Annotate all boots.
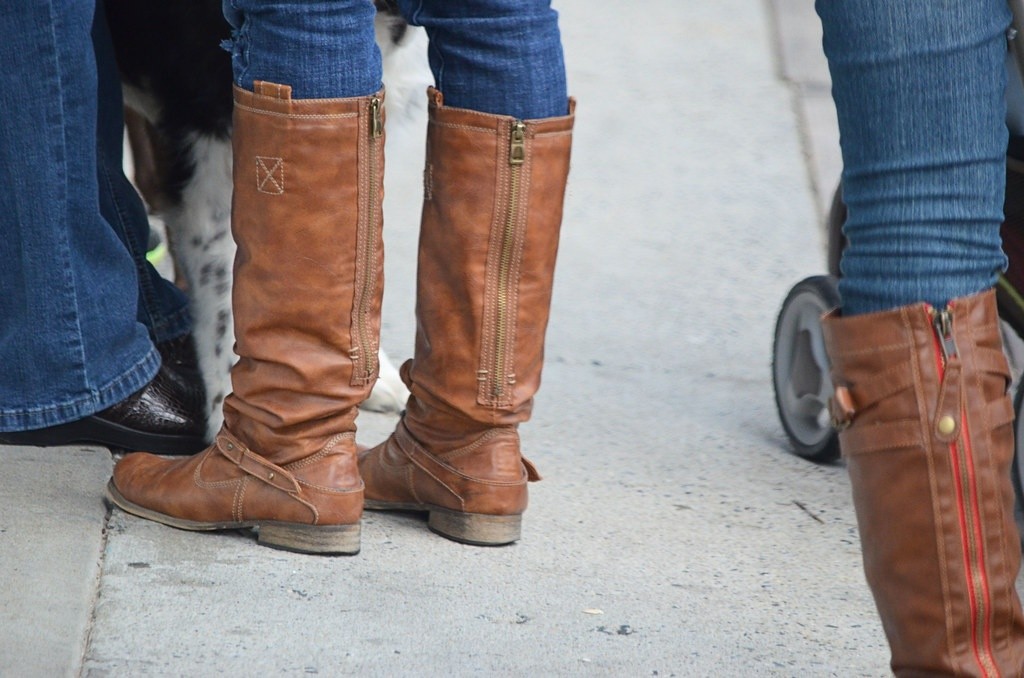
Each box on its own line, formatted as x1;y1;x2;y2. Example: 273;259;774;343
352;84;576;544
105;83;392;555
817;288;1021;673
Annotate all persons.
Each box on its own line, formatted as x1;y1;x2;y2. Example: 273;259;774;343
105;0;578;558
807;0;1024;678
0;0;209;457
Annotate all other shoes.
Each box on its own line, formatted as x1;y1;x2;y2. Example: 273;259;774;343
154;335;204;370
0;365;209;456
